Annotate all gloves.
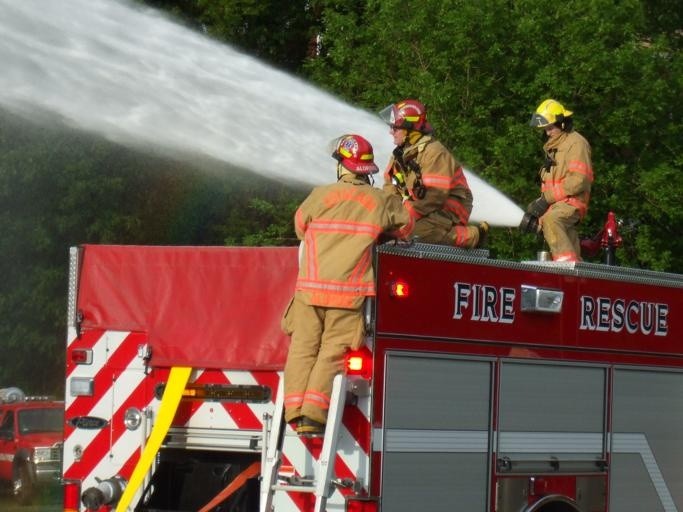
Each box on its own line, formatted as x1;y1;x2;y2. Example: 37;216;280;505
526;192;549;219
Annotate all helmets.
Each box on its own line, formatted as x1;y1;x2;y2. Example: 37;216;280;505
387;99;433;134
534;98;575;129
335;133;380;176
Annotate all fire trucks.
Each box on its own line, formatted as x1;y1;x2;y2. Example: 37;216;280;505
0;387;64;512
63;211;683;511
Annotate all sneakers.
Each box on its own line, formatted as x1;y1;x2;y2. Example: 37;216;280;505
474;221;489;250
294;415;325;433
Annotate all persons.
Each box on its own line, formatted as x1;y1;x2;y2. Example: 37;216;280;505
377;98;489;253
279;133;415;431
525;98;593;265
